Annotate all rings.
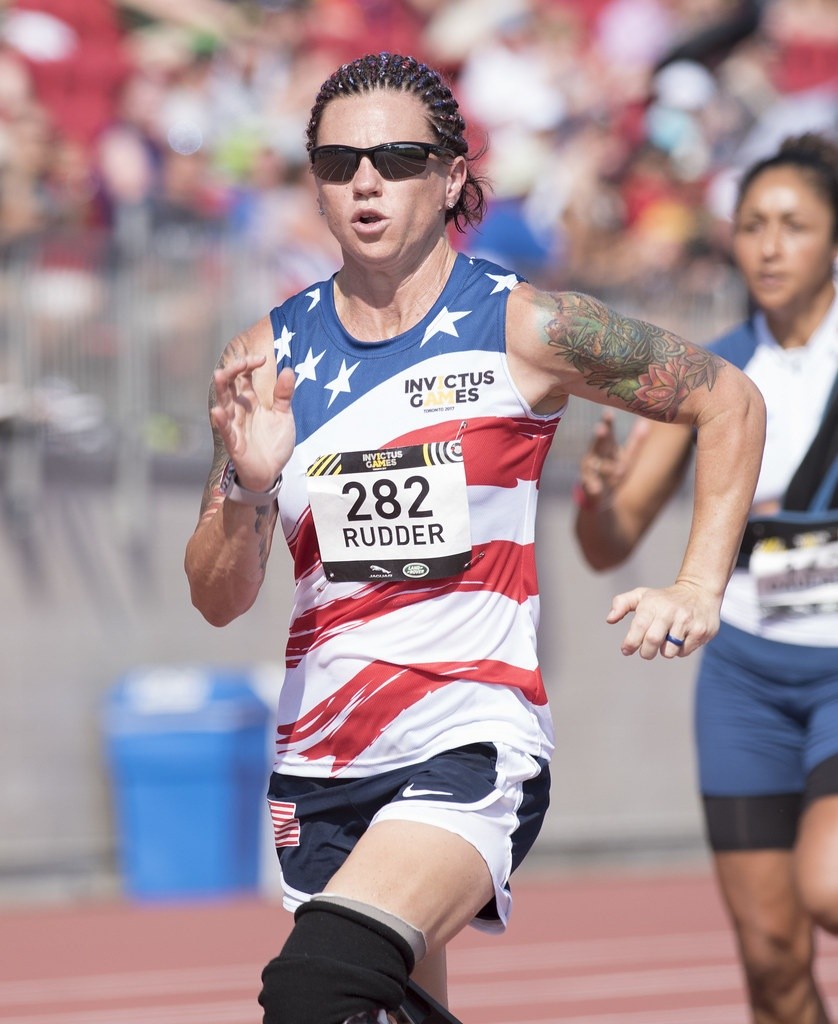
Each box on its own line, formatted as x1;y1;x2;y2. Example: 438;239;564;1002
666;634;683;646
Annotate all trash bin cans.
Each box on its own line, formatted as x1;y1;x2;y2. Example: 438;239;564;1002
103;664;273;903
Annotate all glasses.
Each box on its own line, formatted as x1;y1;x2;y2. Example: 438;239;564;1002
309;140;459;181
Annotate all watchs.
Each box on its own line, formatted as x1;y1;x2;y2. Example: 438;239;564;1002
218;457;283;505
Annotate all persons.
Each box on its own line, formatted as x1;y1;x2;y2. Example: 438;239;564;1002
185;51;766;1024
576;133;837;1024
